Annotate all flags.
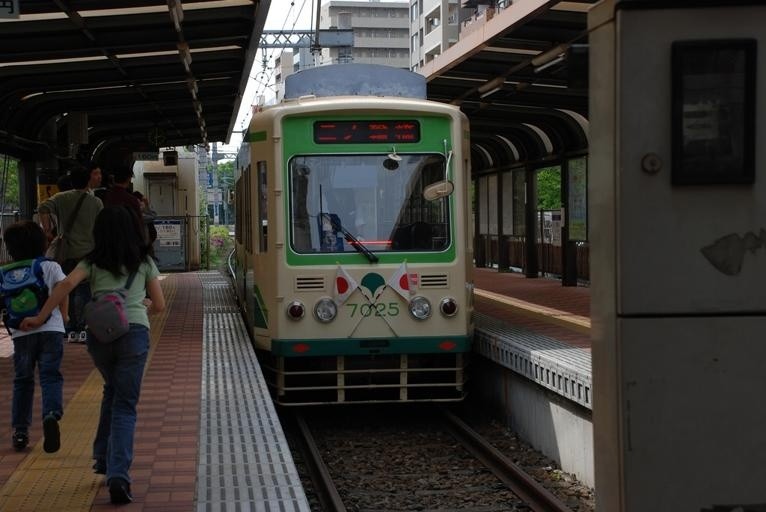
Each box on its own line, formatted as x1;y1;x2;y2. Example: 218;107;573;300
387;261;417;304
333;265;358;306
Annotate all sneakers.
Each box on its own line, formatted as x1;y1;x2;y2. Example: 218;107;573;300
67;329;88;344
12;431;29;452
43;414;60;453
93;458;132;503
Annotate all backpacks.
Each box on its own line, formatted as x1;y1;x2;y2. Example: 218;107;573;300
0;259;51;328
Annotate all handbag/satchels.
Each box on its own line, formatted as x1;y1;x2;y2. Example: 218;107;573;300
45;234;65;265
83;289;131;345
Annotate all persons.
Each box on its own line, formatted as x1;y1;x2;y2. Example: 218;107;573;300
0;220;72;453
89;167;161;264
37;167;104;344
18;205;167;502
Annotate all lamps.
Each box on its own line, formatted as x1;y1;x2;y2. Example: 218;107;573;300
449;27;569;106
166;0;212;153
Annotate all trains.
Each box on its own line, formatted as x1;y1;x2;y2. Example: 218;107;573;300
235;61;480;410
